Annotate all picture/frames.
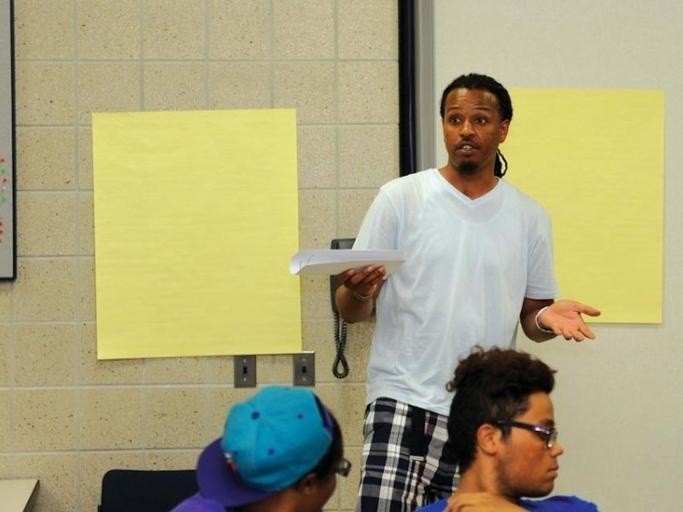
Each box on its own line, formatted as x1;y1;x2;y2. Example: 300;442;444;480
0;0;16;282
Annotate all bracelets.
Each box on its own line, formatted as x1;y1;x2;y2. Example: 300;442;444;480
534;305;562;334
352;292;372;303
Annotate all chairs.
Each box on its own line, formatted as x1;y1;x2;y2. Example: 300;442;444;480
96;469;201;512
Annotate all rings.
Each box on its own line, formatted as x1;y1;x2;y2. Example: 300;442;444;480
343;279;357;289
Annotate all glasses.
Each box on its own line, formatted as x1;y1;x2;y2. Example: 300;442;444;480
487;418;559;449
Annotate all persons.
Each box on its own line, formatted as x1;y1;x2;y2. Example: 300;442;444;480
169;385;351;512
413;343;599;512
335;74;603;510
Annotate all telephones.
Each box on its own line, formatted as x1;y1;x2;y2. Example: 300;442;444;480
330;239;376;378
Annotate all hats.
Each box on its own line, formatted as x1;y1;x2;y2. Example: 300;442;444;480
197;383;337;506
